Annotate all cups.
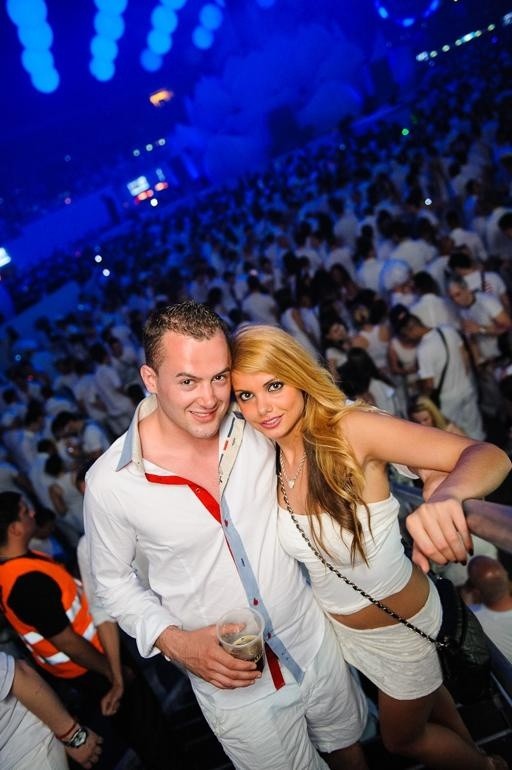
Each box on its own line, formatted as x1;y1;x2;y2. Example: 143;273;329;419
216;607;266;672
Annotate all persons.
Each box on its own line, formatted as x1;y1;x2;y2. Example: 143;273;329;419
0;36;512;558
0;490;182;770
464;553;512;662
76;300;370;770
227;324;512;770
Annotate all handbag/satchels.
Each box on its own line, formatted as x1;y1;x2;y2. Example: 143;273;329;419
412;392;440;409
433;578;490;692
